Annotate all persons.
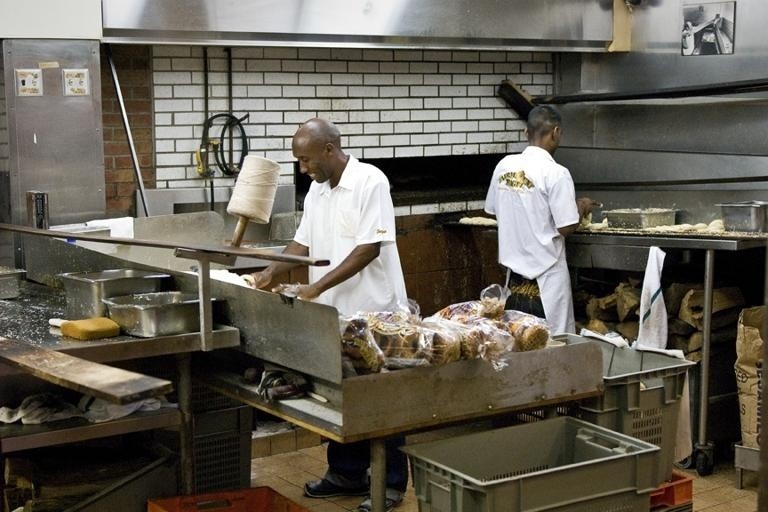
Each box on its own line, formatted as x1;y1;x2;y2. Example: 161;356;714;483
248;118;408;512
683;15;721;55
484;106;600;339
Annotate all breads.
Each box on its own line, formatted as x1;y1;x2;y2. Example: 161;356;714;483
343;319;548;374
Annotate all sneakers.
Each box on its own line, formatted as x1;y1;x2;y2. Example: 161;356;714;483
303;478;370;499
358;497;398;511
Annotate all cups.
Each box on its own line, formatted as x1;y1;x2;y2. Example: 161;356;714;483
26;190;48;229
